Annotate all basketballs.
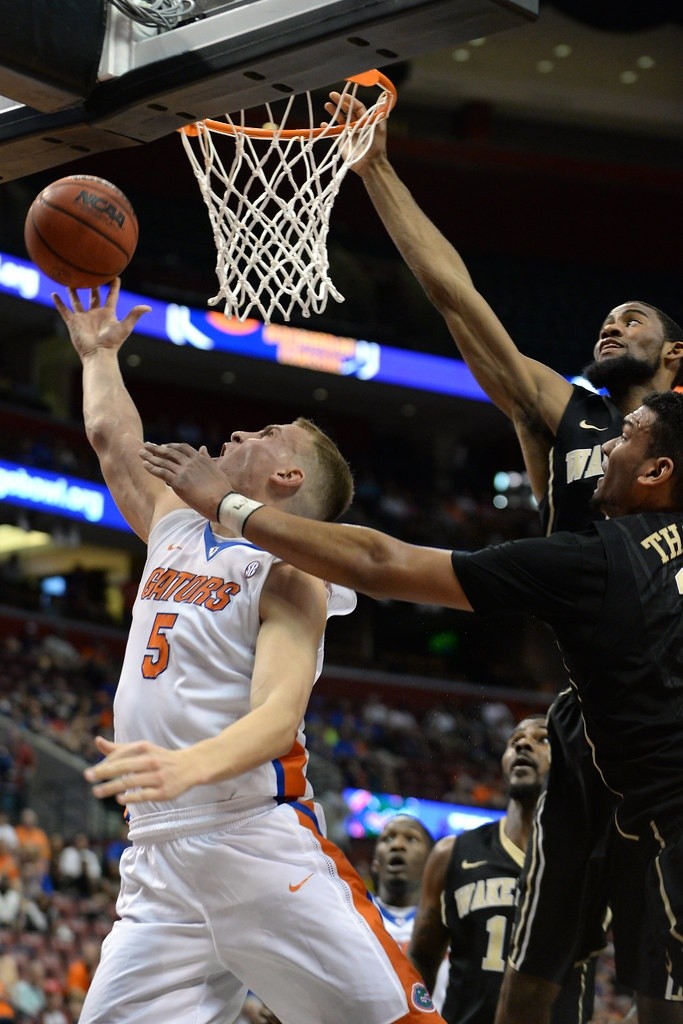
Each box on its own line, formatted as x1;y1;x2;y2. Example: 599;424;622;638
23;173;140;290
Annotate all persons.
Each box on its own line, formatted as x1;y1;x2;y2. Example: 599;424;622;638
137;392;683;1024
318;93;683;539
51;279;446;1024
367;813;451;1016
0;605;683;1024
408;715;597;1024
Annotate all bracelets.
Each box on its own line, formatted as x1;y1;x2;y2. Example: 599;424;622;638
217;491;265;539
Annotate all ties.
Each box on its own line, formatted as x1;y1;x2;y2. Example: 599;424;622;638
77;847;87;876
